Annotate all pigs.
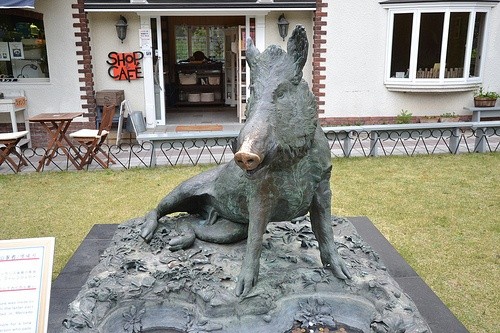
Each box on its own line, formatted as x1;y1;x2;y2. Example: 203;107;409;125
138;22;352;298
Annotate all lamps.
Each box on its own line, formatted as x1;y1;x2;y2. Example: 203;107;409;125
114;13;128;43
277;12;290;40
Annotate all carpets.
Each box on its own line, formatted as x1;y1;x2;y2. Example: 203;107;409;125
176;125;222;131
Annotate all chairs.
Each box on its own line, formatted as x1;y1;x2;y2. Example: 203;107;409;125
0;130;29;173
68;103;117;169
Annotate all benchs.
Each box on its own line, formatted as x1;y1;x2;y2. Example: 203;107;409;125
463;106;500;121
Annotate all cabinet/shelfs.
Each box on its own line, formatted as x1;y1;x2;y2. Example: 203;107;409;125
175;61;225;106
224;24;256;123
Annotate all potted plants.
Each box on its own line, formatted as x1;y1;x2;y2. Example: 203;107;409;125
474;86;500;107
439;111;459;121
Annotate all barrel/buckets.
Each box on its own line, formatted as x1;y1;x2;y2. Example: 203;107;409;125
126;112;147;132
201;93;214;102
189;94;200;102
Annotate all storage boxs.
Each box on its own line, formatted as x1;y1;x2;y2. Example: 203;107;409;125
179;73;197;84
96;89;124;106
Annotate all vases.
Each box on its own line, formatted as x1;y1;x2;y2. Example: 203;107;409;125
419;117;439;122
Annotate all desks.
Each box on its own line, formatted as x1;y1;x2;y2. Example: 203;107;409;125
25;112;86;171
0;95;31;154
